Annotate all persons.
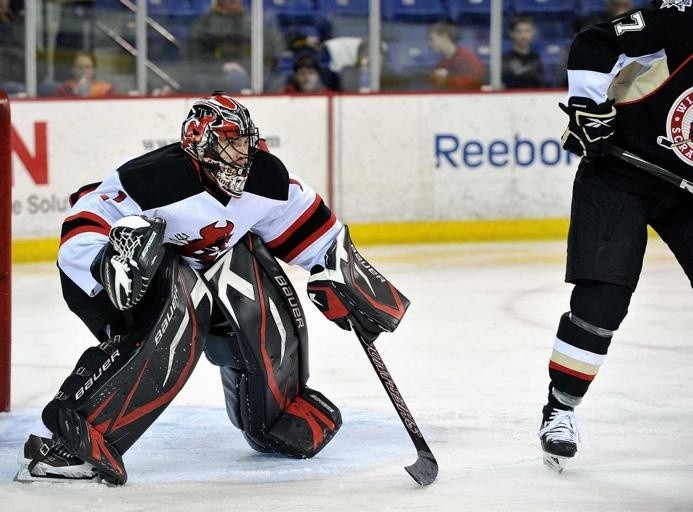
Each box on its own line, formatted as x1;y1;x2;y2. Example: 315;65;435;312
1;1;24;28
536;0;693;460
191;0;341;94
57;51;116;97
15;89;411;474
428;18;544;90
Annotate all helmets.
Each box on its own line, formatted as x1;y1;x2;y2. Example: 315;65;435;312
180;93;260;199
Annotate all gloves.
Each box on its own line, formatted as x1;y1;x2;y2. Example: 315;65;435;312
558;97;626;166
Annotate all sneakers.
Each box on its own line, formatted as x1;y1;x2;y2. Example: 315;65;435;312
17;435;101;478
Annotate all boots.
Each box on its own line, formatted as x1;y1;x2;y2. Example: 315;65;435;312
540;381;586;458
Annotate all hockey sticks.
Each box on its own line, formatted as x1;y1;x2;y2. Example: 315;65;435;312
354;327;439;486
